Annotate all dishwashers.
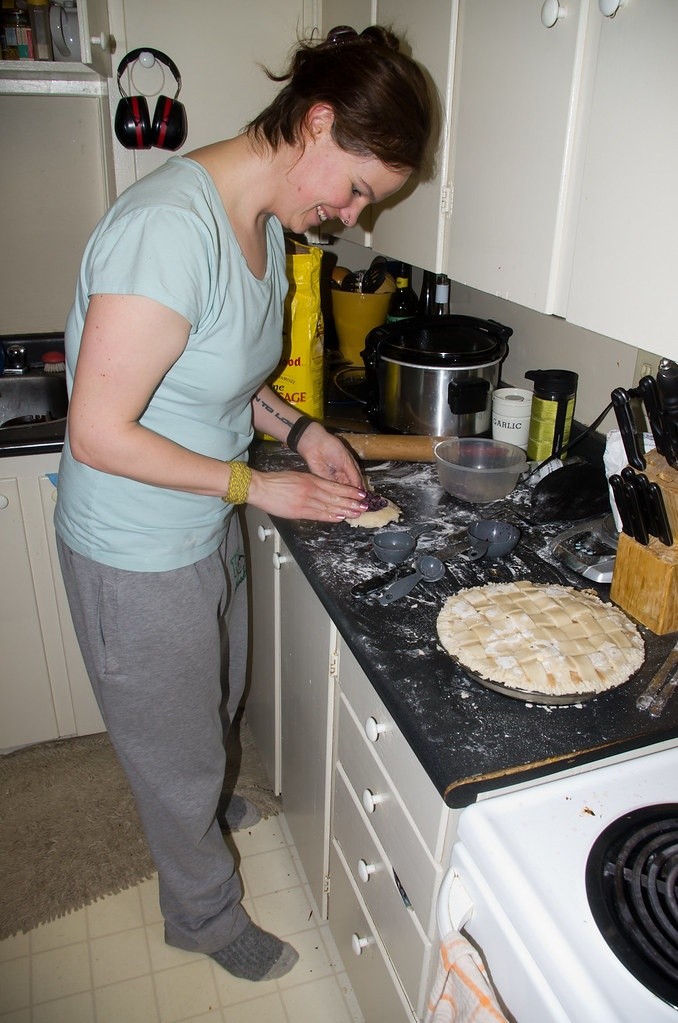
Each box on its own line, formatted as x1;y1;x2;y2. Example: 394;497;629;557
422;841;571;1023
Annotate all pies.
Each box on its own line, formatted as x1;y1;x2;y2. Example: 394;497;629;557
436;579;646;696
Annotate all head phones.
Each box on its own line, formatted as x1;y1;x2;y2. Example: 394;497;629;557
113;47;188;151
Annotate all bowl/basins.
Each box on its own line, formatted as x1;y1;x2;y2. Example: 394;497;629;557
433;438;530;503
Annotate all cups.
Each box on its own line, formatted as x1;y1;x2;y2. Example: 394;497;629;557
492;386;532;453
50;1;81;62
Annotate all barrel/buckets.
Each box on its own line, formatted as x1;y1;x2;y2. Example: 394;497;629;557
330;287;392;368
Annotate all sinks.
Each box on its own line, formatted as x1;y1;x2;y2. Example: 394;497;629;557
0;375;71;438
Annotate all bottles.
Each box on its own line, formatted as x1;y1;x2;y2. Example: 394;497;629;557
526;369;577;460
387;262;449;321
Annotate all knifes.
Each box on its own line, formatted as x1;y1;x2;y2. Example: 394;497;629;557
350;540;472;598
610;357;678;546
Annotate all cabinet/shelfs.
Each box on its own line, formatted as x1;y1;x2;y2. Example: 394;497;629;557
0;474;60;752
442;1;677;364
38;477;107;737
239;503;337;920
124;1;320;244
320;1;458;274
327;629;449;1022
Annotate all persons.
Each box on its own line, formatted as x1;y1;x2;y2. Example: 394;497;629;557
54;26;443;983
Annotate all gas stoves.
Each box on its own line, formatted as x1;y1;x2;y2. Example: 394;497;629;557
456;734;678;1023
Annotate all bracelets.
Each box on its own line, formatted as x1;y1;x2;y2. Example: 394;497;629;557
287;415;317;453
221;460;251;505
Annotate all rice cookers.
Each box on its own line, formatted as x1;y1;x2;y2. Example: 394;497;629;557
360;315;513;436
